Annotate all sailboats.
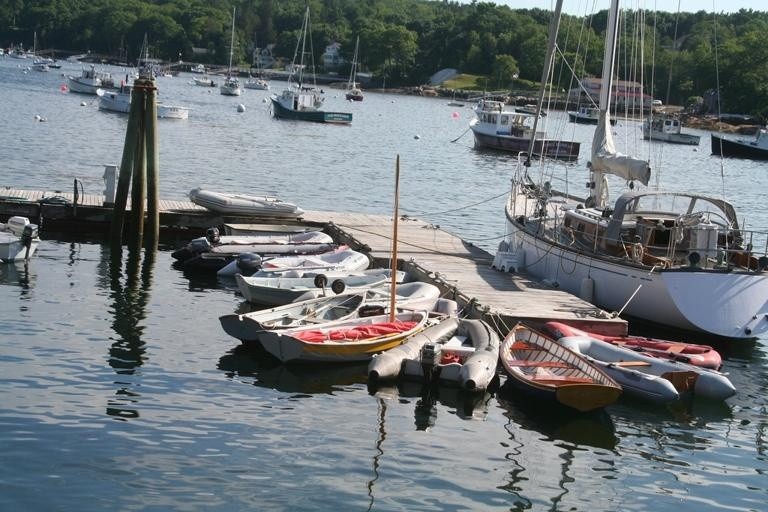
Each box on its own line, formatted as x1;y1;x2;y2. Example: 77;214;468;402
505;0;766;341
346;35;364;101
283;6;325;108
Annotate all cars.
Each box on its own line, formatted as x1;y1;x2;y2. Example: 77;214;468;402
653;100;662;105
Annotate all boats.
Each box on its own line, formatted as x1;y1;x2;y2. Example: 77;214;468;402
639;108;700;145
514;104;547;119
367;315;501;393
0;216;40;262
182;236;341;264
545;320;723;372
270;92;353;123
192;76;219;87
31;62;50;71
468;99;581;164
189;187;305;218
216;248;369;279
500;321;624;412
218;282;440;343
26;50;35;58
170;231;339;260
251;250;368;278
10;46;27;58
68;62;122;95
710;126;768;161
223;223;326;237
32;55;54;64
232;273;394;307
556;336;738;404
568;104;618;126
221;72;241;95
256;309;429;364
244;77;270;90
45;61;61;69
96;30;189;119
